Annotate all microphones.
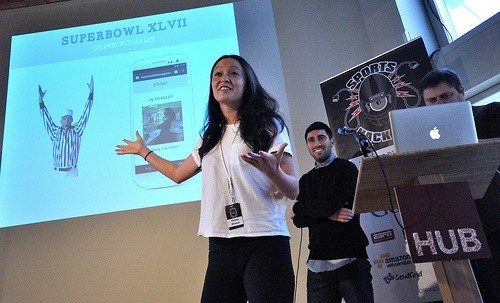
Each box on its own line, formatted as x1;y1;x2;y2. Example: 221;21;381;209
341;124;348;136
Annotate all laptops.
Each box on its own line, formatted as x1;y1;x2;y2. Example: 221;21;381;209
389;100;479;153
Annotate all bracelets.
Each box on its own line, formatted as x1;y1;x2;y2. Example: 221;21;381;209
145;151;154;161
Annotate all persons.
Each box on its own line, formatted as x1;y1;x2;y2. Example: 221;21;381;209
38;75;94;178
116;55;300;303
418;69;500;303
290;122;374;303
